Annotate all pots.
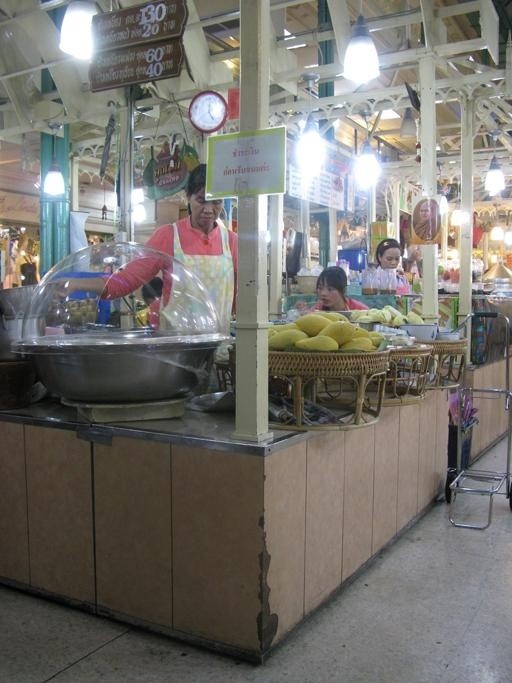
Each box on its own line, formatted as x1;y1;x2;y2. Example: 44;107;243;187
0;313;42;361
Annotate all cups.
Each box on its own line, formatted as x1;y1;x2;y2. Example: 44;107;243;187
380;270;397;294
361;271;378;295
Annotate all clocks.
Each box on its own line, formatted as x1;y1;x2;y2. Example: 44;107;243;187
189;90;228;133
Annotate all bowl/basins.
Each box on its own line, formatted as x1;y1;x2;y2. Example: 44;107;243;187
0;282;39;315
297;274;319;294
399;322;439;344
437;329;462;344
10;325;229;404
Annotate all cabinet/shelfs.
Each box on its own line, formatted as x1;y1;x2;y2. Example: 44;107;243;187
1;351;451;655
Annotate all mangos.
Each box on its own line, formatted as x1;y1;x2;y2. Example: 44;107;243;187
268;310;386;353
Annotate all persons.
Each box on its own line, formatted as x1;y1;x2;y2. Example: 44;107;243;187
309;267;368;312
15;250;42;288
362;238;411;295
134;278;166;325
413;201;439;239
47;164;239;335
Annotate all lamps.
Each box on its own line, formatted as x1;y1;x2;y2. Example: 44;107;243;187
484;139;504;196
399;110;418;141
451;176;467;226
437;166;449;216
504;230;512;247
342;1;380;84
59;2;101;62
353;118;382;190
43;135;65;197
295;88;325;169
133;190;147;223
490;204;504;241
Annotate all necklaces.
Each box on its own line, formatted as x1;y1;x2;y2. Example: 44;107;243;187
23;255;33;265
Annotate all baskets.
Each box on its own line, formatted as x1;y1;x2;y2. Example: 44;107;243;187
367;347;434;409
269;349;391;431
418;339;469;392
